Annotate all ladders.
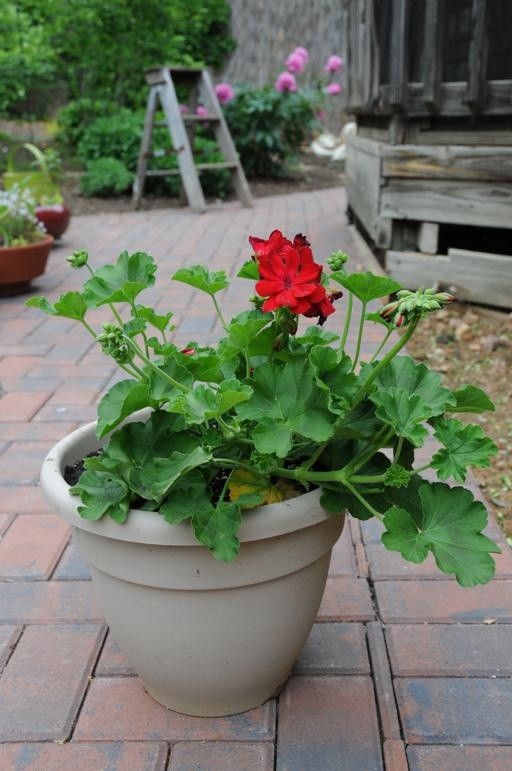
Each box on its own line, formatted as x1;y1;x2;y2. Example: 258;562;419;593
130;64;255;215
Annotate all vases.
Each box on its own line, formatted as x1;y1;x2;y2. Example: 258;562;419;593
39;405;346;719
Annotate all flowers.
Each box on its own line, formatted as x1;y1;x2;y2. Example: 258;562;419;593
166;45;344;199
20;225;503;589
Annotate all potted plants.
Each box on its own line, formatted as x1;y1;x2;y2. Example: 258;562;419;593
0;143;71;297
